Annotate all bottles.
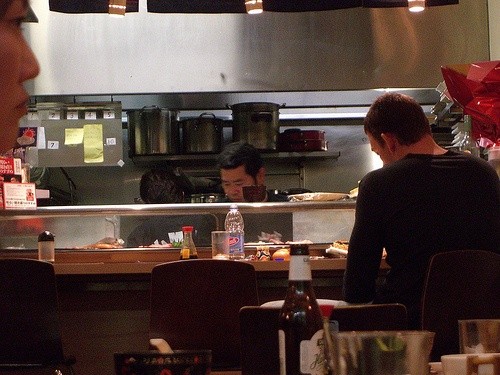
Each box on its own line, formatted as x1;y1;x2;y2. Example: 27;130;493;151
279;244;330;374
458;114;500;182
179;226;198;259
38;231;55;264
225;204;245;259
251;246;290;260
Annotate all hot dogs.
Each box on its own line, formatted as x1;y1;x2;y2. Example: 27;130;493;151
332;240;349;250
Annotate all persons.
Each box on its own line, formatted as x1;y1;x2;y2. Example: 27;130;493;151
338;90;500;358
130;141;296;246
0;174;20;190
0;0;42;152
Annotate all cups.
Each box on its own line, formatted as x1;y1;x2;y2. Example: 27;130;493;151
332;330;434;375
211;231;230;260
430;319;500;375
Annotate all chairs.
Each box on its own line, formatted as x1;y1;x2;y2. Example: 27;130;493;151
236;304;411;375
150;259;260;352
421;251;500;349
0;258;63;367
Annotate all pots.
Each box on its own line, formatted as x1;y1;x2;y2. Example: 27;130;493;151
126;104;178;156
226;101;286;151
179;111;234;153
280;128;328;152
189;192;228;202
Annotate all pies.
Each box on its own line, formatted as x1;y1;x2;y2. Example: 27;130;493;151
94;243;122;249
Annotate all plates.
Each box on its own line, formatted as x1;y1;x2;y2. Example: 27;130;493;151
288;190;347;201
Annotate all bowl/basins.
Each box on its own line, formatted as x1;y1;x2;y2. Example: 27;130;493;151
242;184;267;202
268;189;288;202
112;350;213;375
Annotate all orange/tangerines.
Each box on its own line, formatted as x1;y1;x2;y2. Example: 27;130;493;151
272;249;290;260
